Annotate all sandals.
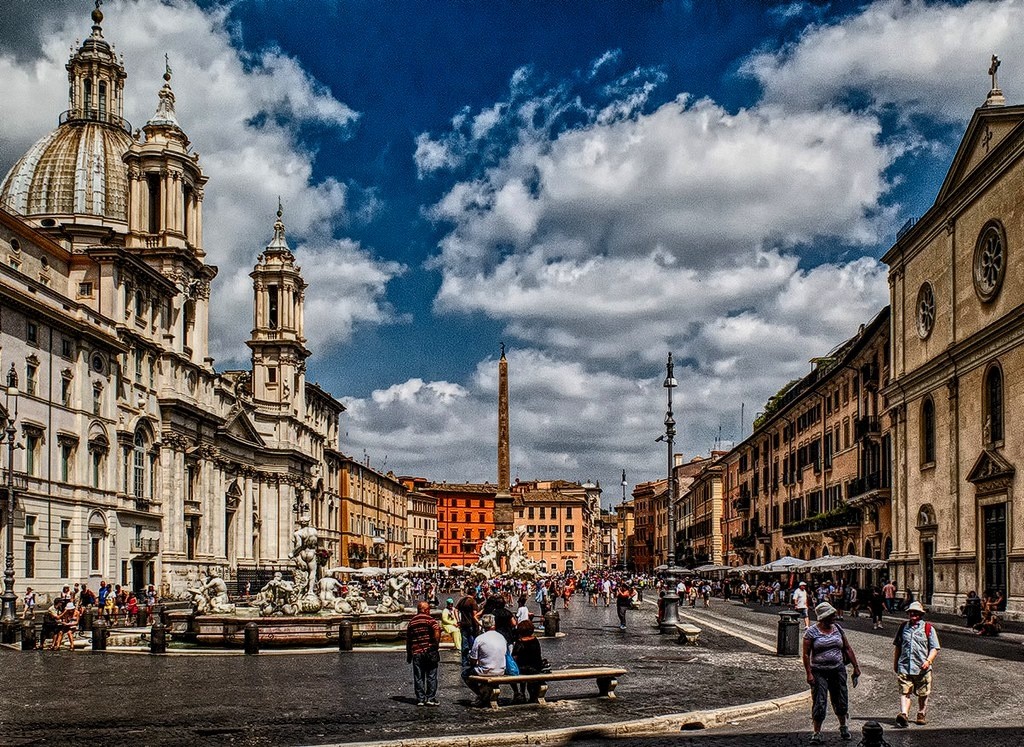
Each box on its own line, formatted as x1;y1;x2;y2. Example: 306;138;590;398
11;615;159;651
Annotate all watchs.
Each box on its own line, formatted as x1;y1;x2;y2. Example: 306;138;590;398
852;664;860;668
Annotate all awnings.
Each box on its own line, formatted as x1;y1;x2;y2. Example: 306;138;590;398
653;549;888;586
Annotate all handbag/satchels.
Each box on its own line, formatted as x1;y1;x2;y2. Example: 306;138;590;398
408;622;440;662
505;650;519;676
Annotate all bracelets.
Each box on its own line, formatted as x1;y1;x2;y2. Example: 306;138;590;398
925;658;932;666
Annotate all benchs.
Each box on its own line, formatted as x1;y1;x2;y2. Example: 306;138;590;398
673;623;702;646
510;609;560;637
467;666;628;712
3;612;447;655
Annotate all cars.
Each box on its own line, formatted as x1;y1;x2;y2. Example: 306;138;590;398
347;581;362;589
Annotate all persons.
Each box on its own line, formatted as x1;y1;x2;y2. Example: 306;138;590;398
474;523;540;581
404;601;444;706
254;512;412;613
980;592;993;612
961;589;981;627
987;590;1006;611
891;599;941;726
458;611;511;710
324;565;915;664
244;579;251;600
200;568;240;615
507;616;546;708
972;609;1002;637
801;601;861;746
20;577;161;653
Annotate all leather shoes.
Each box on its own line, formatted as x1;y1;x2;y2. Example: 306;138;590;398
896;712;908;726
916;710;926;725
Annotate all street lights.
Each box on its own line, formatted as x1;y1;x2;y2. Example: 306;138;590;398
386;505;391;581
0;361;27;630
540;541;544;572
653;351;692;635
621;468;628;581
461;532;466;571
292;500;310;517
436;525;440;574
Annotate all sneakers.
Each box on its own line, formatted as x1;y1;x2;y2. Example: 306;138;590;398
511;692;522;703
418;697;425;705
810;731;824;745
838;725;851;740
470;695;484;706
518;694;527;703
426;696;441;705
619;623;627;629
538;625;546;629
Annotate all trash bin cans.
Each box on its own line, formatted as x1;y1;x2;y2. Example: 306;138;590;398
777;610;801;657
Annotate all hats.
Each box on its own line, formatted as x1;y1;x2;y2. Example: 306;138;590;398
815;602;837;621
905;601;926;615
799;582;807;586
